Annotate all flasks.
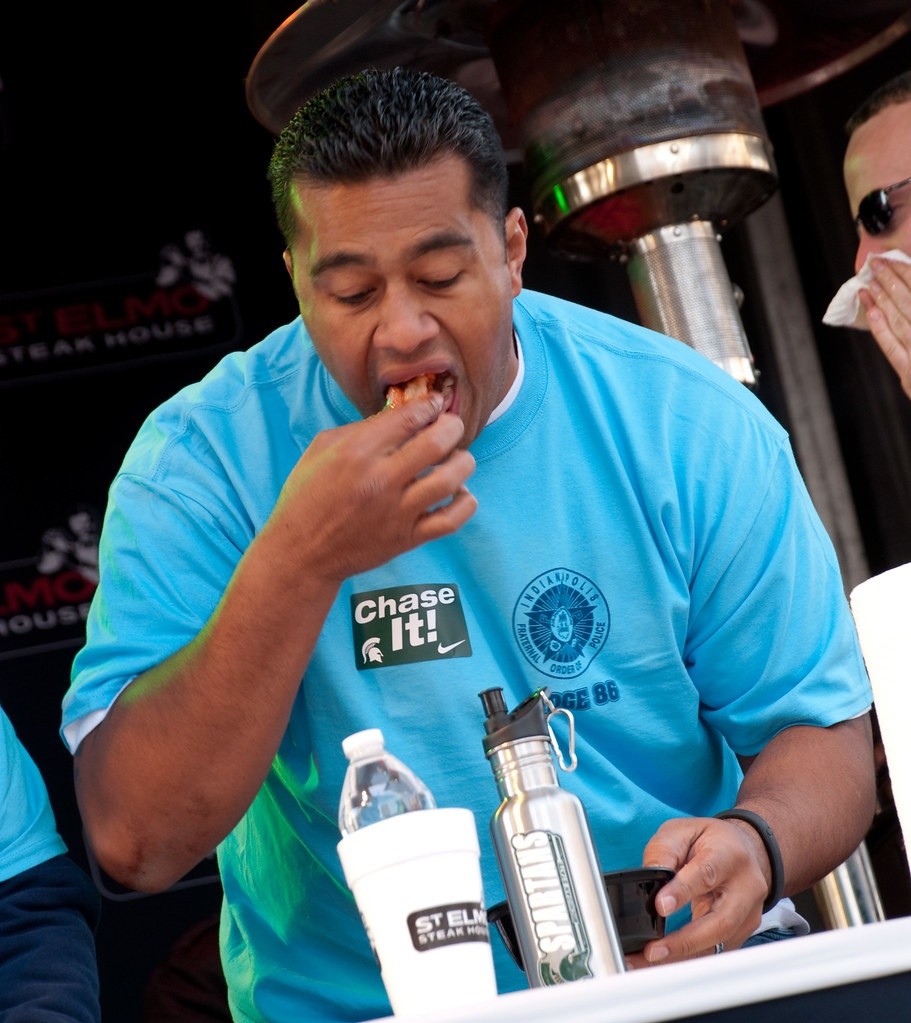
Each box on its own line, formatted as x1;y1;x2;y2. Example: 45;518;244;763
477;681;626;988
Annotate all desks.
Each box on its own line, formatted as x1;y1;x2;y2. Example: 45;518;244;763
360;912;911;1023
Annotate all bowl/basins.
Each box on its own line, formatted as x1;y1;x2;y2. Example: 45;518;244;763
486;868;673;972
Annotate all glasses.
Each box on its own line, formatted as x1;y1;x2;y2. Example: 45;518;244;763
853;176;911;236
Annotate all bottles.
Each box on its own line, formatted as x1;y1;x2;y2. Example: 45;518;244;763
336;730;435;839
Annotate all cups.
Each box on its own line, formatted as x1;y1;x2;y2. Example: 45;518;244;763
335;806;496;1023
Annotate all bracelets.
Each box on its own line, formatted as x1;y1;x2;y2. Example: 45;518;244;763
713;808;785;914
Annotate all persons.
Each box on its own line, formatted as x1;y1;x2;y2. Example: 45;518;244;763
0;707;100;1023
841;68;911;403
54;60;877;1023
